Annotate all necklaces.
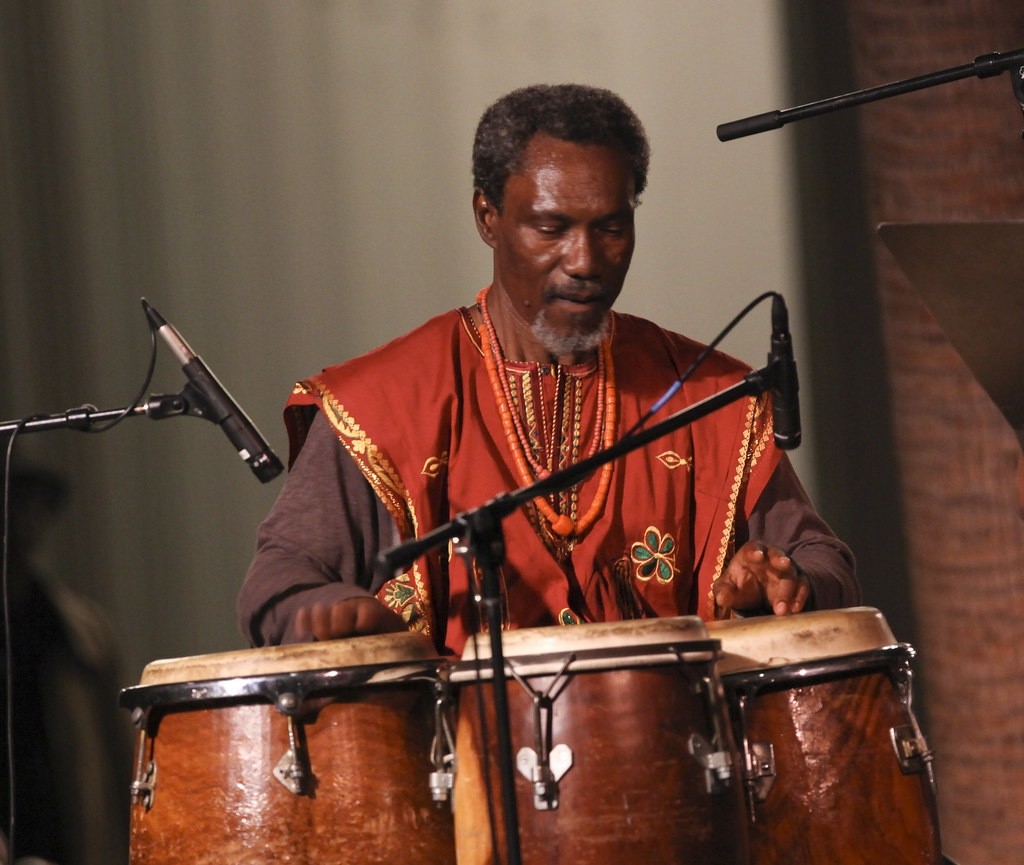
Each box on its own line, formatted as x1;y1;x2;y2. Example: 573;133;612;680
477;285;618;563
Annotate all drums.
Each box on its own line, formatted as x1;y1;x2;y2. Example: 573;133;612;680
127;631;454;865
450;615;755;865
704;605;945;865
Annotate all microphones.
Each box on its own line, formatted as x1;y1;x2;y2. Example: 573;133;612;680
145;304;284;484
769;294;801;451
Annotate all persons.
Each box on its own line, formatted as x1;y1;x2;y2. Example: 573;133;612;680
235;85;861;648
